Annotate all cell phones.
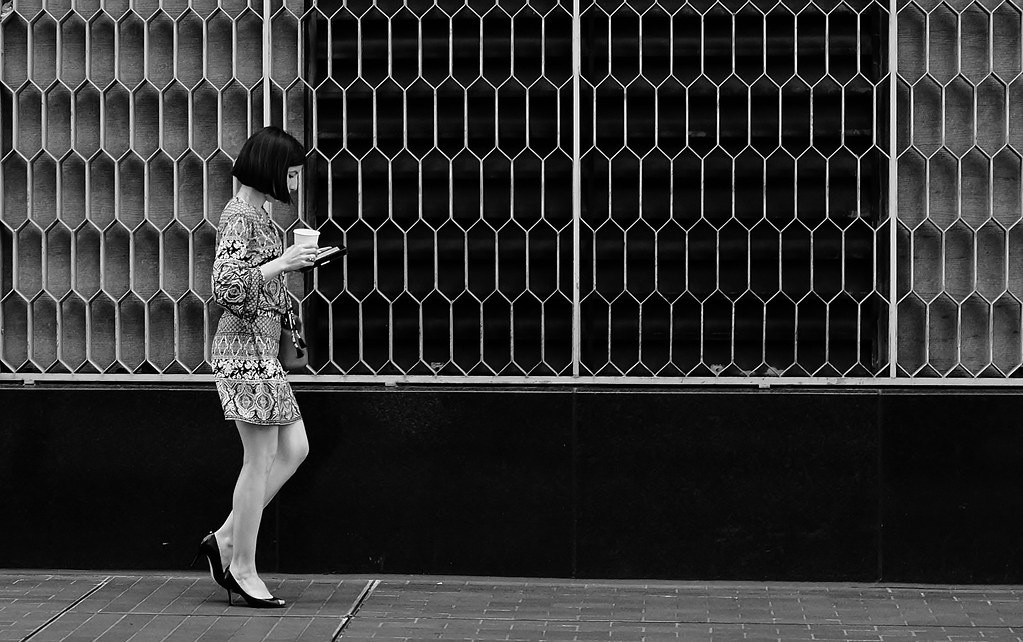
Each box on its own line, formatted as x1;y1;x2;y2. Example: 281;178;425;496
300;244;348;273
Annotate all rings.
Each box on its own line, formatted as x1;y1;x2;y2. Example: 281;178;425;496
308;254;313;260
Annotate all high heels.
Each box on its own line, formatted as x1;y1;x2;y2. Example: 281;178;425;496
190;531;226;589
223;569;286;608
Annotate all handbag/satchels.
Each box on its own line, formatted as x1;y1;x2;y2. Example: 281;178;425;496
278;325;308;370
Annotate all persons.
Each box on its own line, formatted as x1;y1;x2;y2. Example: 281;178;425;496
193;124;321;607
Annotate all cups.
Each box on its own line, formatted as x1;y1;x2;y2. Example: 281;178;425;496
293;228;320;250
